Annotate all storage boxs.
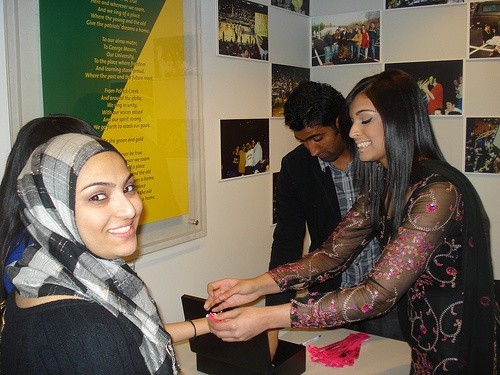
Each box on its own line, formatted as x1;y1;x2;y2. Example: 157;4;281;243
180;295;306;375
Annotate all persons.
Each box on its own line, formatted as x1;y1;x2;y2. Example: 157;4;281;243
1;132;179;375
0;115;227;344
204;67;500;375
265;79;407;360
219;0;500;177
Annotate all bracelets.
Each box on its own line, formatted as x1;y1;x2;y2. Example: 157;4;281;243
190;320;196;339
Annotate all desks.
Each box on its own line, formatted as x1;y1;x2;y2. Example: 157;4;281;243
172;326;413;375
